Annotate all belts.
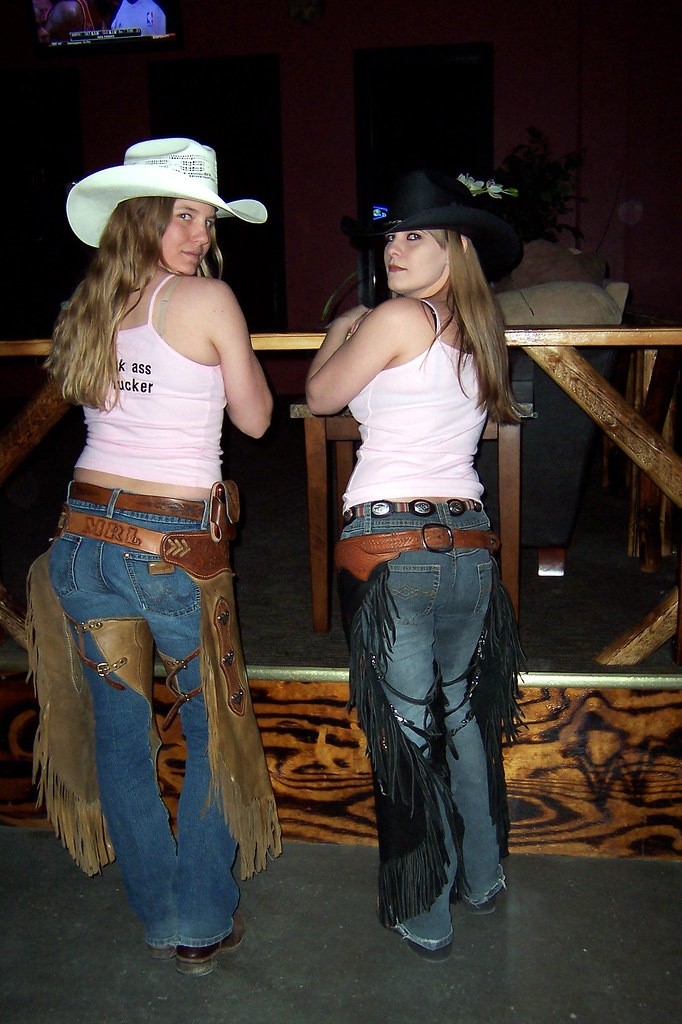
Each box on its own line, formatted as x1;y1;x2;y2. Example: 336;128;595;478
326;526;498;581
68;480;206;521
54;504;231;578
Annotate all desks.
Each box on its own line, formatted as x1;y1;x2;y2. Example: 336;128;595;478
287;379;537;638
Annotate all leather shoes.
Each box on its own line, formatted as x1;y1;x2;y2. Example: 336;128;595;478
176;910;248;975
375;899;453;963
464;896;497;914
147;941;177;959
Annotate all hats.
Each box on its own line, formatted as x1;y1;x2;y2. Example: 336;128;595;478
66;138;269;248
338;171;525;284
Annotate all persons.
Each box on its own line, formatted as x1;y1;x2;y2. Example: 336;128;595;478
46;130;278;974
305;160;531;956
